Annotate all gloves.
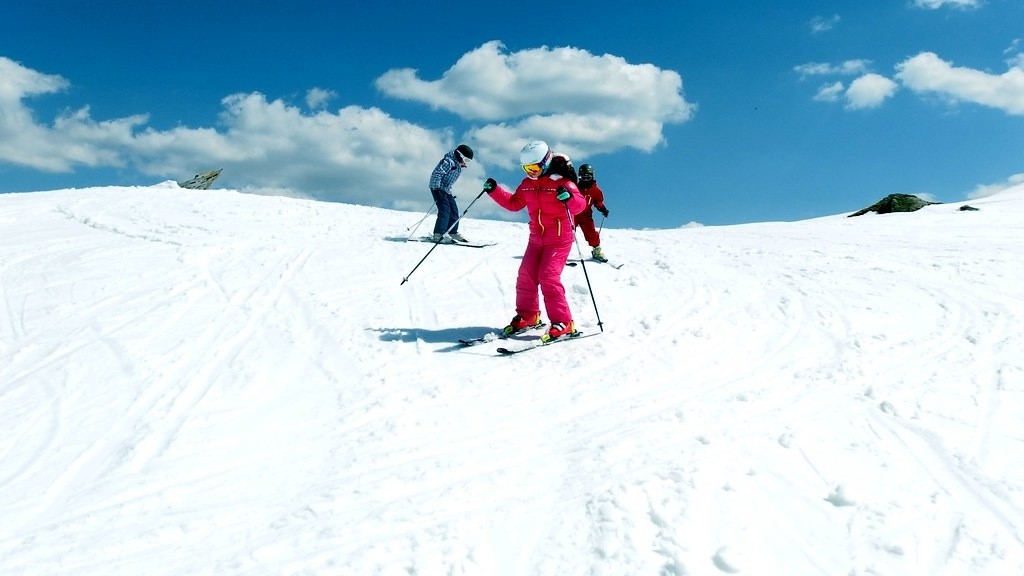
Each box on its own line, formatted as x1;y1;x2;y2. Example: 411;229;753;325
556;186;573;202
433;190;442;200
600;206;609;217
484;178;497;194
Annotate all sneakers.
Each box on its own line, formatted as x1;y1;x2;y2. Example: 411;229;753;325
502;314;542;335
434;232;457;244
593;247;608;262
448;234;468;243
542;320;577;342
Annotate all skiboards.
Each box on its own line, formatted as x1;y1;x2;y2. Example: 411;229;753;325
568;257;623;269
457;322;581;355
386;235;498;248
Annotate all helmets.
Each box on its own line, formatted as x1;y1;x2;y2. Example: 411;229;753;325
578;164;593;177
454;145;474;163
520;140;552;166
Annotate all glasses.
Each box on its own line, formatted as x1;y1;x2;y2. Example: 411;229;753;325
457;150;472;165
580;174;592;180
523;149;551;173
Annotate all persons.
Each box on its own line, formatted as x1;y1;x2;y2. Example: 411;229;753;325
483;141;584;341
430;145;474;242
575;164;609;261
